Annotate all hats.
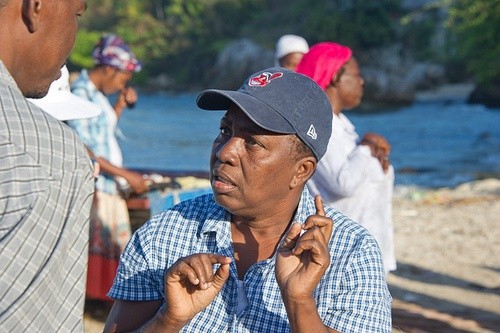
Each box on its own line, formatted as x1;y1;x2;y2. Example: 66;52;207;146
24;64;102;120
95;34;142;72
274;35;310;67
294;42;352;88
195;66;333;162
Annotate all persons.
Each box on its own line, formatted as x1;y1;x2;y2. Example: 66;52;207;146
295;42;397;272
0;0;95;333
275;35;309;71
64;32;149;265
104;66;393;333
